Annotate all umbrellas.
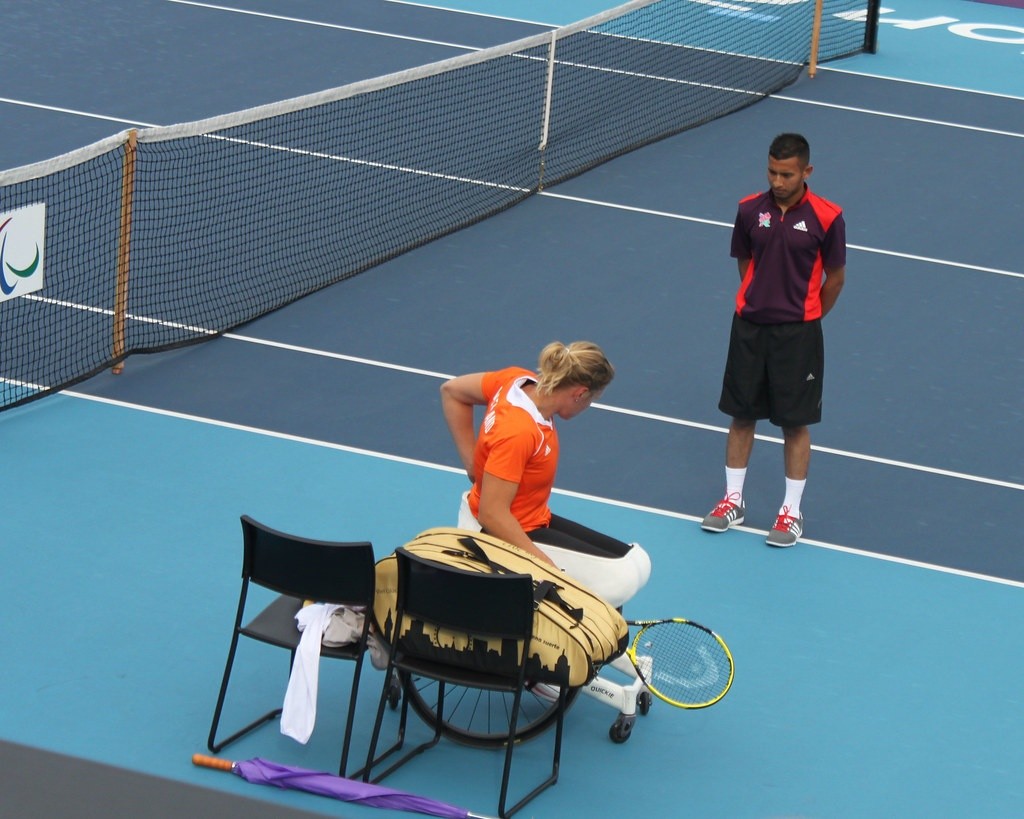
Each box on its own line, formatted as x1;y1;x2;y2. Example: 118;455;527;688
189;753;491;818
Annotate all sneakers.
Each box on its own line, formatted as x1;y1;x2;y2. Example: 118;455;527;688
701;486;745;532
766;504;803;547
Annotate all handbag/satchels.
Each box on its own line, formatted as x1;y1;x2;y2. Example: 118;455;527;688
374;527;630;688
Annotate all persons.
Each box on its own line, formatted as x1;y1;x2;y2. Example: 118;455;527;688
440;341;639;611
700;134;847;549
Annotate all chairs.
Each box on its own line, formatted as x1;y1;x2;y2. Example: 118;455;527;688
364;546;568;819
208;515;406;783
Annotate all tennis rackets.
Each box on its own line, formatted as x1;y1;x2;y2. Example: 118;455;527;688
625;620;733;709
